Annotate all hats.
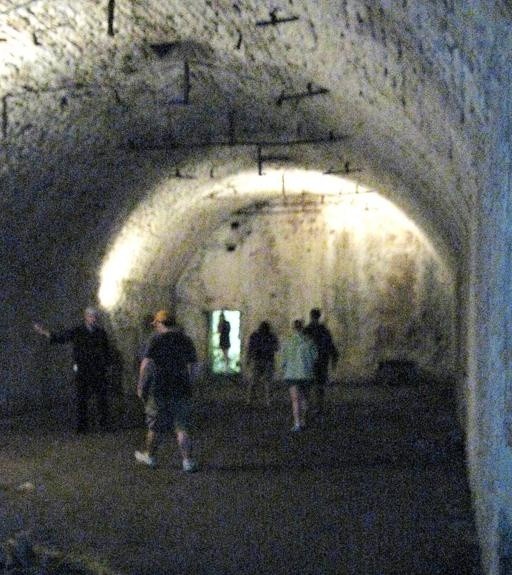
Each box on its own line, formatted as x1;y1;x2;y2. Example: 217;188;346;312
149;310;174;327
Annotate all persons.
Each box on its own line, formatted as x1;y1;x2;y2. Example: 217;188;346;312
282;320;319;433
33;306;111;431
215;314;231;363
132;309;198;474
303;308;338;398
243;321;280;407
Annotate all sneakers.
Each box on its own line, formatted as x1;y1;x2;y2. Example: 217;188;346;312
134;450;159;467
182;457;199;472
